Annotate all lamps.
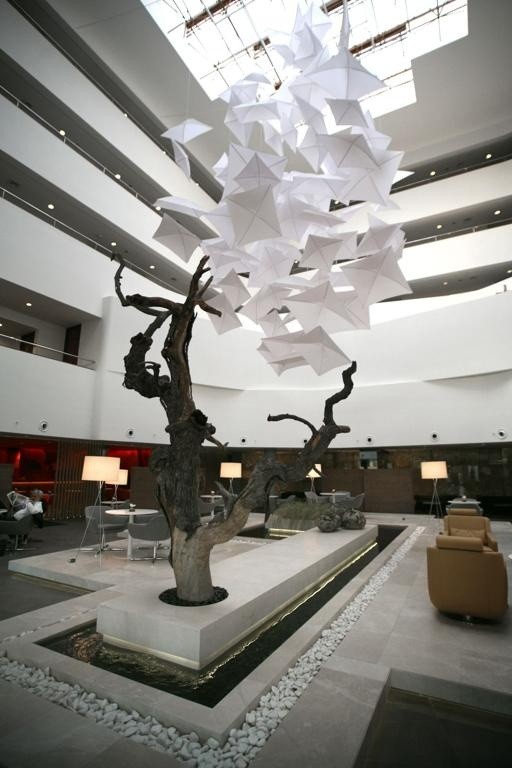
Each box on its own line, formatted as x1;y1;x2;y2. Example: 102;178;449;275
219;461;243;493
303;463;322;498
419;460;448;518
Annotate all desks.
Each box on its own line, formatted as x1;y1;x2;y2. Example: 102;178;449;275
104;508;159;559
319;491;351;507
198;494;223;521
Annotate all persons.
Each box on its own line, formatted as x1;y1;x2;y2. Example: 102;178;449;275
7;489;46;547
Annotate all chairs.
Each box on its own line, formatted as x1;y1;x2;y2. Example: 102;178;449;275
71;455;129;568
427;515;509;623
129;513;171;564
0;503;37;551
345;493;365;511
304;491;320;506
